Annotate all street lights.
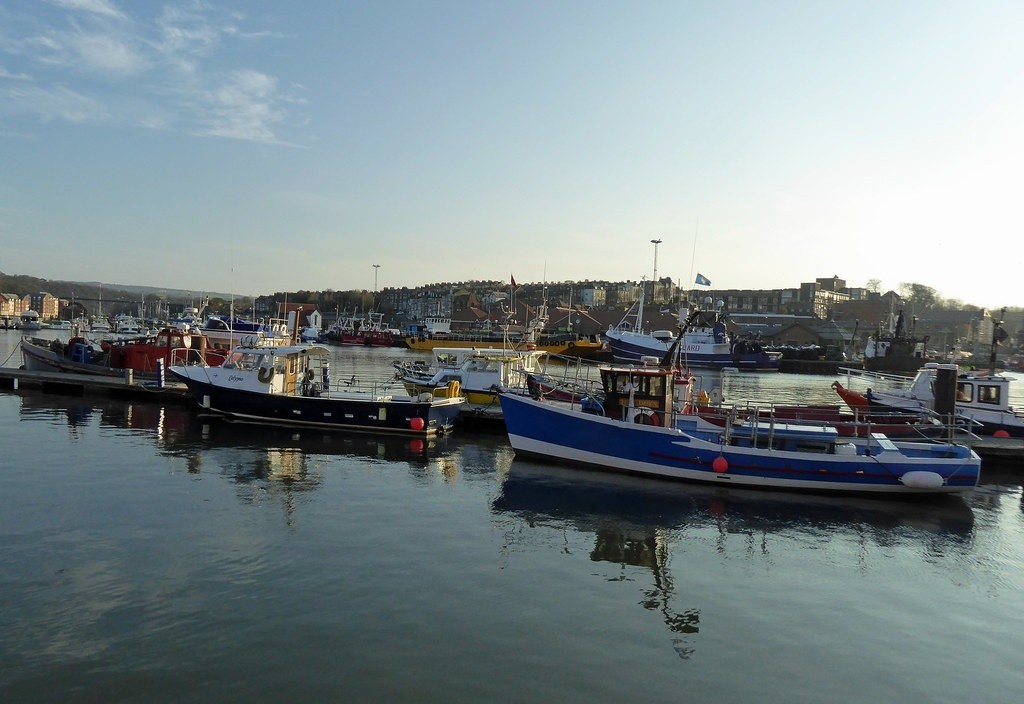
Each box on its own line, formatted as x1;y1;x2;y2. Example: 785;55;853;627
372;264;381;310
650;239;667;303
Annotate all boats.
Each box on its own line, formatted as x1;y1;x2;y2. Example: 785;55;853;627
867;310;933;376
391;346;982;496
300;296;782;371
21;313;295;379
14;282;265;334
835;363;1024;438
165;336;469;441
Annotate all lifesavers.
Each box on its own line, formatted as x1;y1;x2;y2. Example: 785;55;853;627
550;341;554;346
545;341;549;346
539;342;543;346
568;342;575;349
560;341;565;345
258;367;275;384
309;369;315;380
411;339;415;344
446;380;459;398
418;338;425;342
555;341;560;346
625;406;659;426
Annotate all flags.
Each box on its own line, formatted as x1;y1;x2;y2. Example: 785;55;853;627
695;274;711;286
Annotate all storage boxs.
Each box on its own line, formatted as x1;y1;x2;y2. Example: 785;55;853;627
580;397;603;417
72;342;91;364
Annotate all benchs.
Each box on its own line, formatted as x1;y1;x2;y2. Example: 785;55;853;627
320;392;393;400
871;432;899;452
